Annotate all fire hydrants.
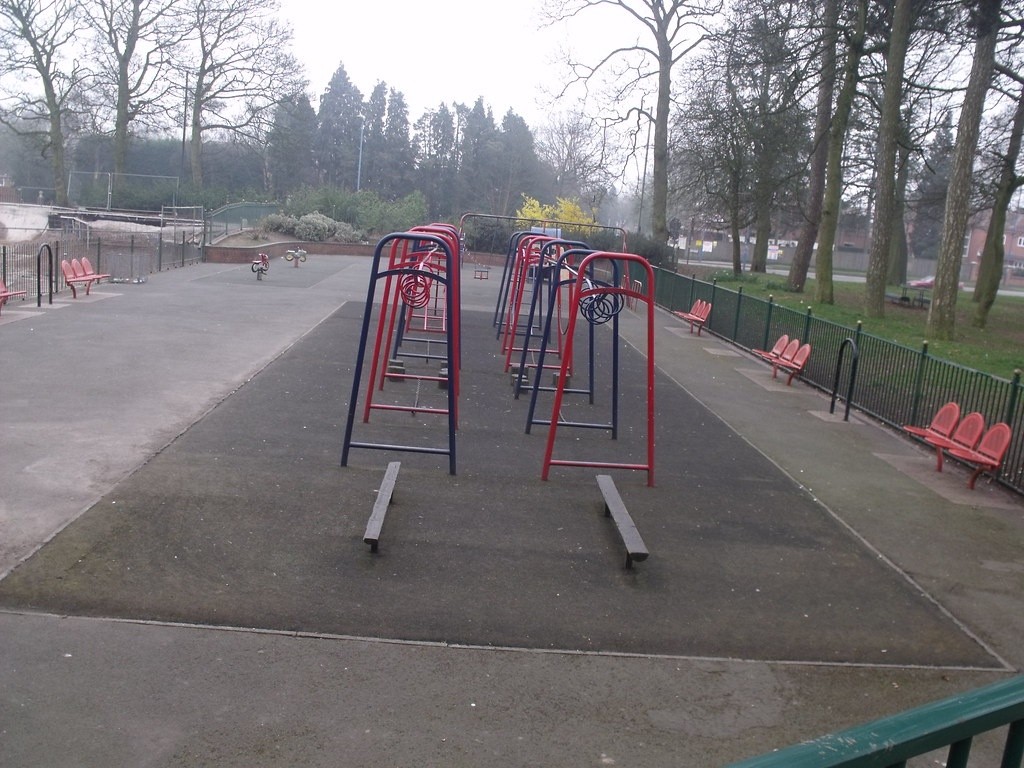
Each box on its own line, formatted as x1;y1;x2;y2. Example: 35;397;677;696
292;246;302;269
256;265;268;281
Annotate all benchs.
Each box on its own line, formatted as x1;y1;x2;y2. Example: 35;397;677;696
596;474;649;570
61;256;111;299
0;272;27;316
885;292;911;305
904;403;1011;489
914;297;931;308
363;460;401;553
752;335;811;385
672;300;712;337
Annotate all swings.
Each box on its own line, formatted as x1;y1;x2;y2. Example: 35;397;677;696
472;218;499;281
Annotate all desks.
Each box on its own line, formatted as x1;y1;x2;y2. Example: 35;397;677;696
899;283;929;309
